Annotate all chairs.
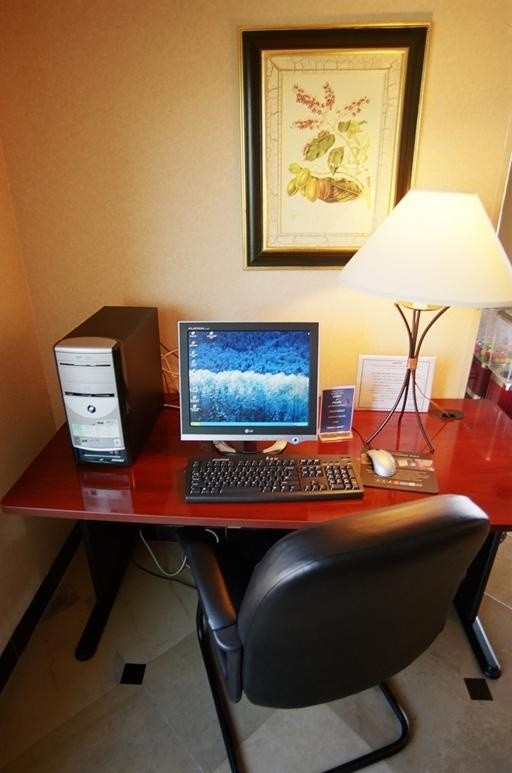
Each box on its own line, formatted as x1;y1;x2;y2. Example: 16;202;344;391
171;488;491;773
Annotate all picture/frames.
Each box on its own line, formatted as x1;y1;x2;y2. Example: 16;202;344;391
235;16;437;273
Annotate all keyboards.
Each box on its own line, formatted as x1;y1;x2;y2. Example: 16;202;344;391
185;457;365;504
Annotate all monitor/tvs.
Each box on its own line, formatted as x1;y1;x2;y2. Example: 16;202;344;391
177;320;319;457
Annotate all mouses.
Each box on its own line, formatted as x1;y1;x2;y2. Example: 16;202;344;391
366;448;397;477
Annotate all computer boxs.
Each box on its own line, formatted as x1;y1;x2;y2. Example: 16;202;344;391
52;306;164;468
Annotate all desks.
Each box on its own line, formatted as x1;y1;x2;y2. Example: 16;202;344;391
1;394;510;685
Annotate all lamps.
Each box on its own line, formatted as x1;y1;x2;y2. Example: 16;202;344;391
329;186;511;452
